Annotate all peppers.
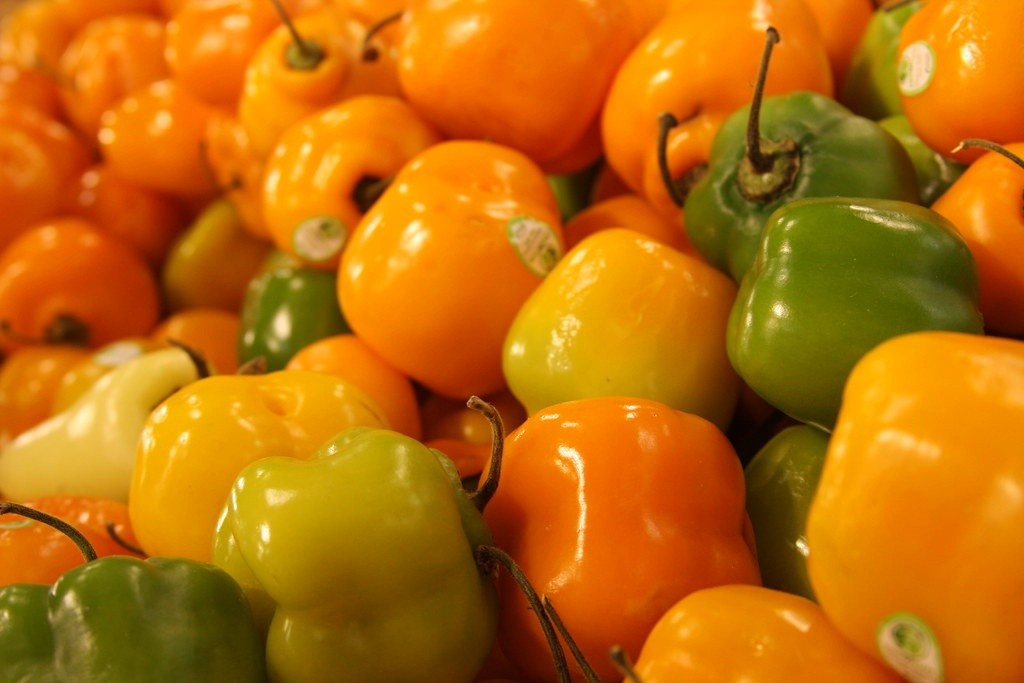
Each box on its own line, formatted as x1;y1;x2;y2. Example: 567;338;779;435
0;0;1024;683
